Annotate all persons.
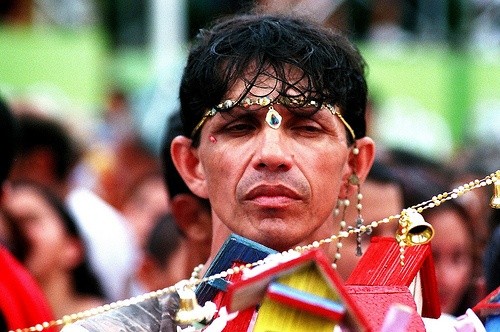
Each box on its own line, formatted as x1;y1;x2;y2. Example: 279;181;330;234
0;0;500;332
60;13;376;332
